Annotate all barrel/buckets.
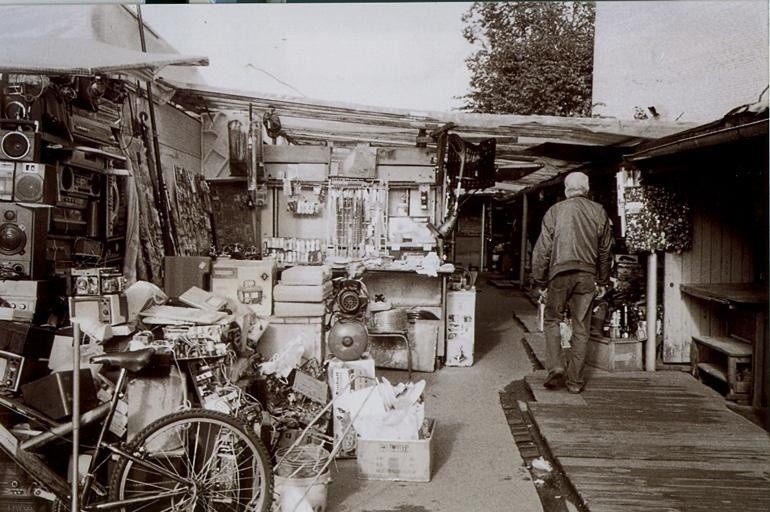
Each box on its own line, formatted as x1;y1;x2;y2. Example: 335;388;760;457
272;467;330;512
275;446;329;477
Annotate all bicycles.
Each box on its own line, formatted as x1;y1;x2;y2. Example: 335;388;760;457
0;345;281;512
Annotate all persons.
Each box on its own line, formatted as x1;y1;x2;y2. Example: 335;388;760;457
531;170;611;394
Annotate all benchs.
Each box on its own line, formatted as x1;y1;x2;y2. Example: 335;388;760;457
692;335;753;396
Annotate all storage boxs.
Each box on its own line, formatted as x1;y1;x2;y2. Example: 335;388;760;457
356;418;437;483
587;336;643;373
210;259;277;316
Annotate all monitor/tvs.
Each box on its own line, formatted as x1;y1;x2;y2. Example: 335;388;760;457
69;293;127;326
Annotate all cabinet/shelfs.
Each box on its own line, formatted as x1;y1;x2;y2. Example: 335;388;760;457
446;285;476;366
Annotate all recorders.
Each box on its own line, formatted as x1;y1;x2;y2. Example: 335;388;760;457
55;161;103;197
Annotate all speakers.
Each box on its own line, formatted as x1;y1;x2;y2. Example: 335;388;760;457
13;162;56;204
0;130;41;163
0;82;41;130
0;203;48;280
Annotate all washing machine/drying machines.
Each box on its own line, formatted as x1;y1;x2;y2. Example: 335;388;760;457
0;202;35;279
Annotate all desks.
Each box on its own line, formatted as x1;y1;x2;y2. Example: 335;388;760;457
680;282;769;408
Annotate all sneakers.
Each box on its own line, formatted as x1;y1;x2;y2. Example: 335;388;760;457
543;366;566;391
564;374;587;394
